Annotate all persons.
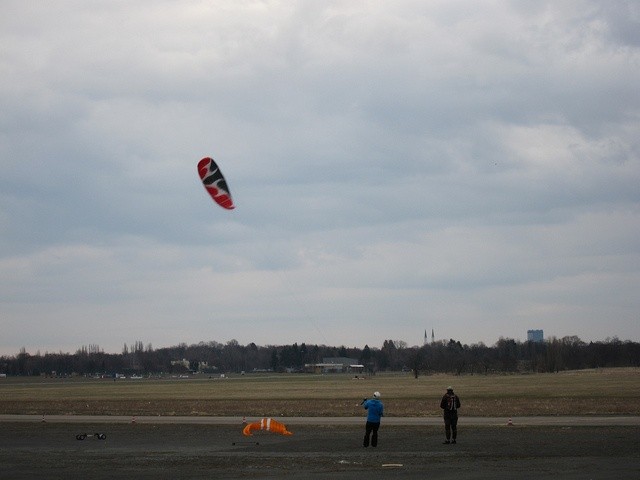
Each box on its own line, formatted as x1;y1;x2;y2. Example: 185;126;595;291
440;386;460;444
362;391;384;448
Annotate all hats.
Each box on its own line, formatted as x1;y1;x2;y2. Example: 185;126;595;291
373;392;380;397
447;386;453;390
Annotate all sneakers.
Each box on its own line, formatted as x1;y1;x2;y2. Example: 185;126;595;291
442;441;450;444
450;440;456;444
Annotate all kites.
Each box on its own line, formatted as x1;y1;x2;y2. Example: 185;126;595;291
197;155;235;214
238;418;293;442
